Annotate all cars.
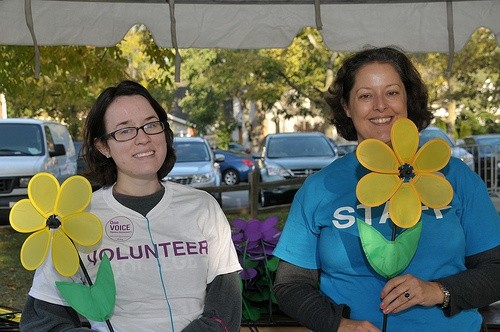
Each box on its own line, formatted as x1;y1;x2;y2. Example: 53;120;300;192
252;131;358;208
213;149;257;185
72;141;102;188
457;133;500;186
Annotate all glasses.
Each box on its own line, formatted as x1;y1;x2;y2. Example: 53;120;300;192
100;120;166;142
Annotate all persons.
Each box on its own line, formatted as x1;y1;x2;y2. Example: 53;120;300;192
19;80;244;332
273;45;492;332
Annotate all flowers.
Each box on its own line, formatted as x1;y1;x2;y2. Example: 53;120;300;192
356;117;456;332
9;172;116;332
229;216;280;322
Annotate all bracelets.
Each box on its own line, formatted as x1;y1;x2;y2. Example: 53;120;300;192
440;284;450;309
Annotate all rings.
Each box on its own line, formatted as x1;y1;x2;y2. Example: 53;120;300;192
405;291;410;301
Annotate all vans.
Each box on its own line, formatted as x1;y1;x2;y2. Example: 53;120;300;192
0;118;78;211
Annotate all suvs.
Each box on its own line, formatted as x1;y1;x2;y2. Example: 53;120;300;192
162;136;226;209
419;125;475;172
